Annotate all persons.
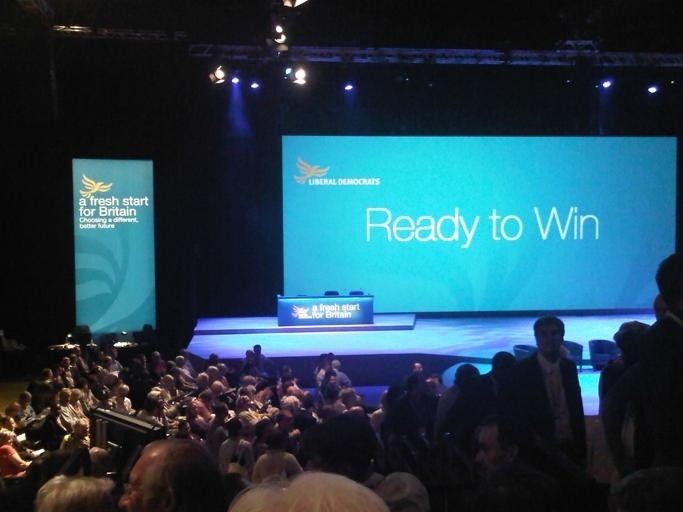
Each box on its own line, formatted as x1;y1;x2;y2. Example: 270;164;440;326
1;323;429;510
381;253;683;510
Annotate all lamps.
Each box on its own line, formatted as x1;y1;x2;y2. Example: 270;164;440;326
207;0;307;90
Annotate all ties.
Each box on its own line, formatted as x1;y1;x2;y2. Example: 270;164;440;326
550;369;560;424
